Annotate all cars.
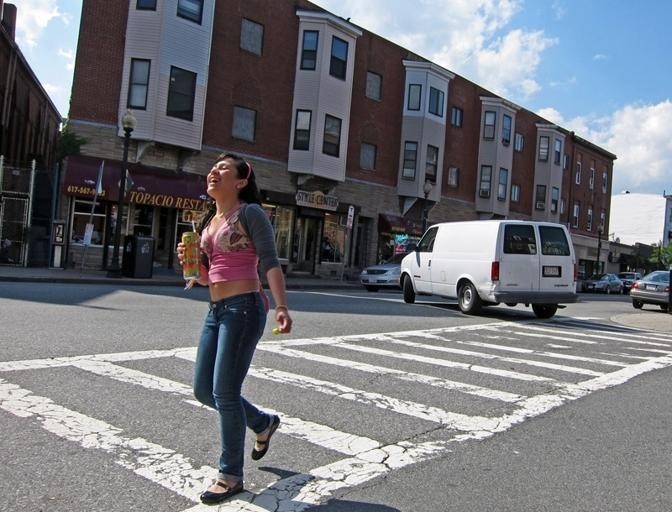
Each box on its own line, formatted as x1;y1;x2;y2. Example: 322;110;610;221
584;270;672;313
360;252;410;292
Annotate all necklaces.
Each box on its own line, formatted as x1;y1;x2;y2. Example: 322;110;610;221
215;203;235;217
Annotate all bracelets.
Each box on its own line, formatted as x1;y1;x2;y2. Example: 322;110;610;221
275;304;288;311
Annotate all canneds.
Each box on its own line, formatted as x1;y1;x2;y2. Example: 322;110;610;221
182;232;199;279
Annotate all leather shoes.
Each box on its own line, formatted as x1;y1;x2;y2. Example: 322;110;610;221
251;413;280;461
200;481;244;504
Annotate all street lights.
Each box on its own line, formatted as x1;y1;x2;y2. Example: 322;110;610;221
596;223;603;274
106;109;137;274
423;180;432;237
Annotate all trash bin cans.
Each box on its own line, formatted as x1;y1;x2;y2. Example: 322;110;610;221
121;234;156;279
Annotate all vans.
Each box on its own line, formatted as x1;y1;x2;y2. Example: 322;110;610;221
400;219;577;318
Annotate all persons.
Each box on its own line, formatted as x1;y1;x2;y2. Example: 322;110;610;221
177;154;292;505
